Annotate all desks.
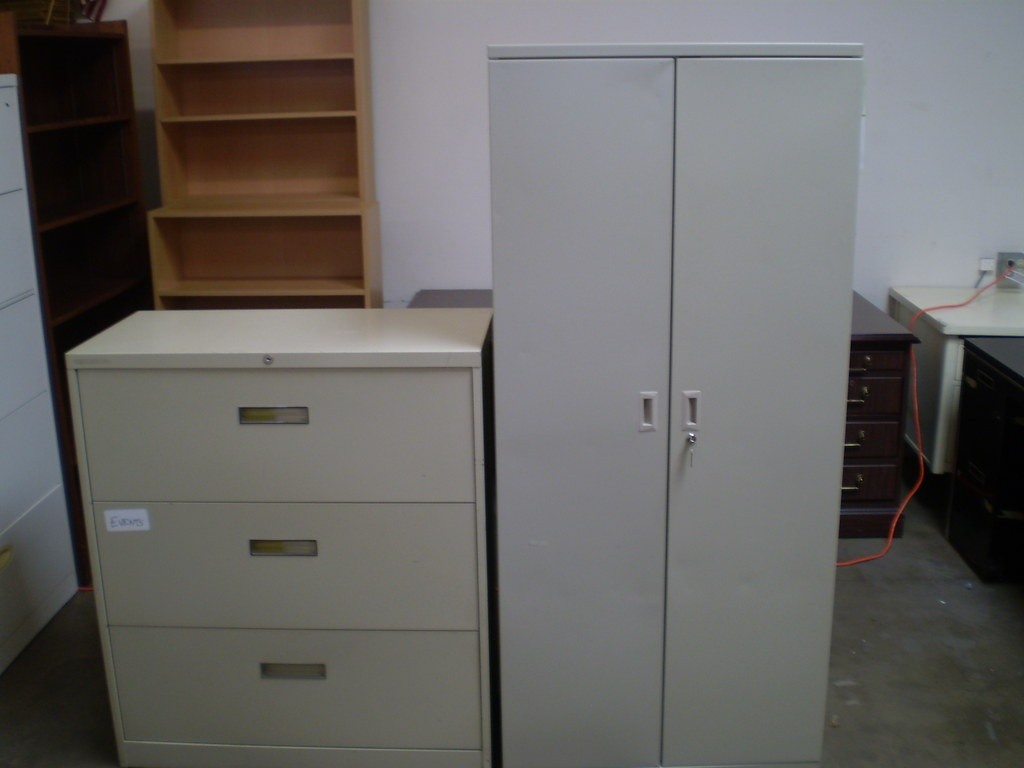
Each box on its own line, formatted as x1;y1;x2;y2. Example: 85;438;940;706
890;285;1024;477
941;337;1024;585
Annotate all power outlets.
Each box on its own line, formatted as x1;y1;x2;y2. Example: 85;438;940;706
995;253;1024;290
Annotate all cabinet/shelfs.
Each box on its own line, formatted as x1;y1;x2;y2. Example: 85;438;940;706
17;20;151;593
62;305;505;768
145;0;388;308
837;291;921;541
486;44;866;768
